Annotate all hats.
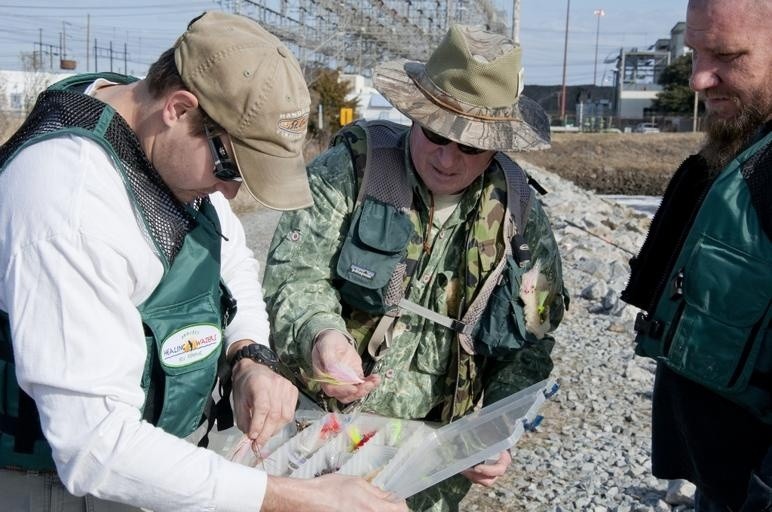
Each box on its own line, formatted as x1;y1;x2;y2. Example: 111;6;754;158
372;23;551;153
174;11;315;213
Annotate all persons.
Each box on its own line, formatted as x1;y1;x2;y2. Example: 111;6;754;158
264;24;570;512
619;0;772;512
0;8;415;512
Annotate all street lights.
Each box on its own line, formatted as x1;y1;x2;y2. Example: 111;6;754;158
591;8;605;104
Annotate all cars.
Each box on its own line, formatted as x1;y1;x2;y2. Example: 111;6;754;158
634;122;660;133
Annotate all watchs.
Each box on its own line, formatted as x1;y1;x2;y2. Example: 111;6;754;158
229;342;279;375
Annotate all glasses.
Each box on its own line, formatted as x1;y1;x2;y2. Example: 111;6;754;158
420;124;486;155
203;123;244;184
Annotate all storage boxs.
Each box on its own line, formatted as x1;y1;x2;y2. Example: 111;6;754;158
240;377;561;503
